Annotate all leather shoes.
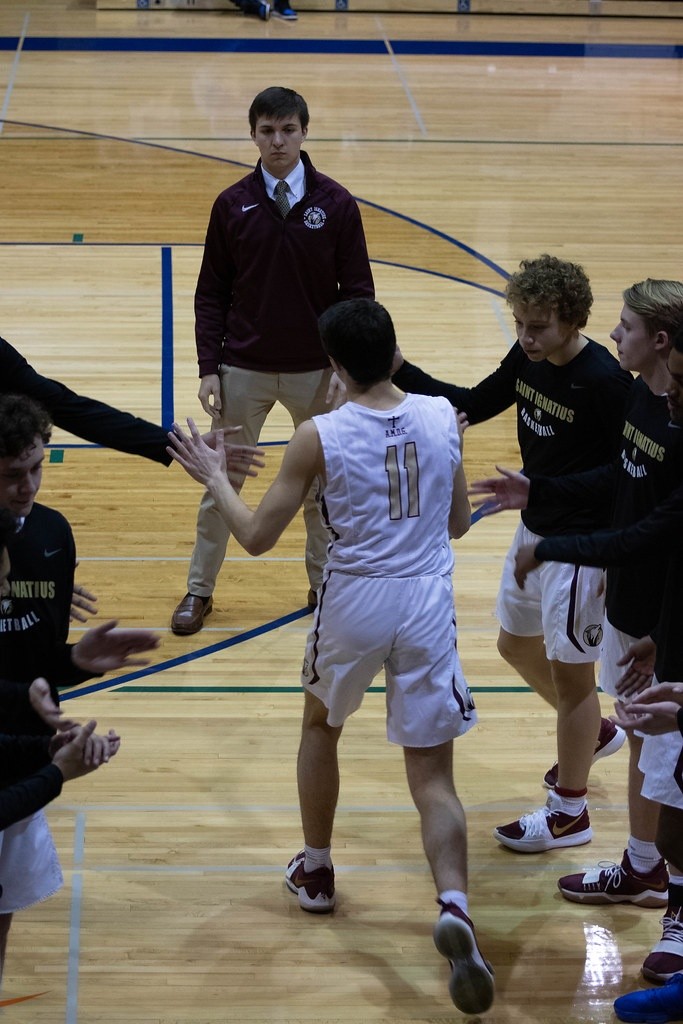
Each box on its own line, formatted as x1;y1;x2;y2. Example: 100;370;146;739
308;588;318;612
171;592;213;636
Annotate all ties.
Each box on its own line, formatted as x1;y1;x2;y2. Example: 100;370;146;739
275;181;290;219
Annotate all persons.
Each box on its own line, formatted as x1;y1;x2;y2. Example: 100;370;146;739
0;338;266;986
462;253;683;1024
232;0;299;21
167;302;497;1012
171;88;375;636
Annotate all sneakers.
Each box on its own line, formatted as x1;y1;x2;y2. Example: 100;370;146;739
557;849;670;908
285;850;336;912
272;6;297;20
543;717;626;789
493;789;592;851
614;973;683;1024
433;898;494;1015
240;0;271;20
641;882;683;986
